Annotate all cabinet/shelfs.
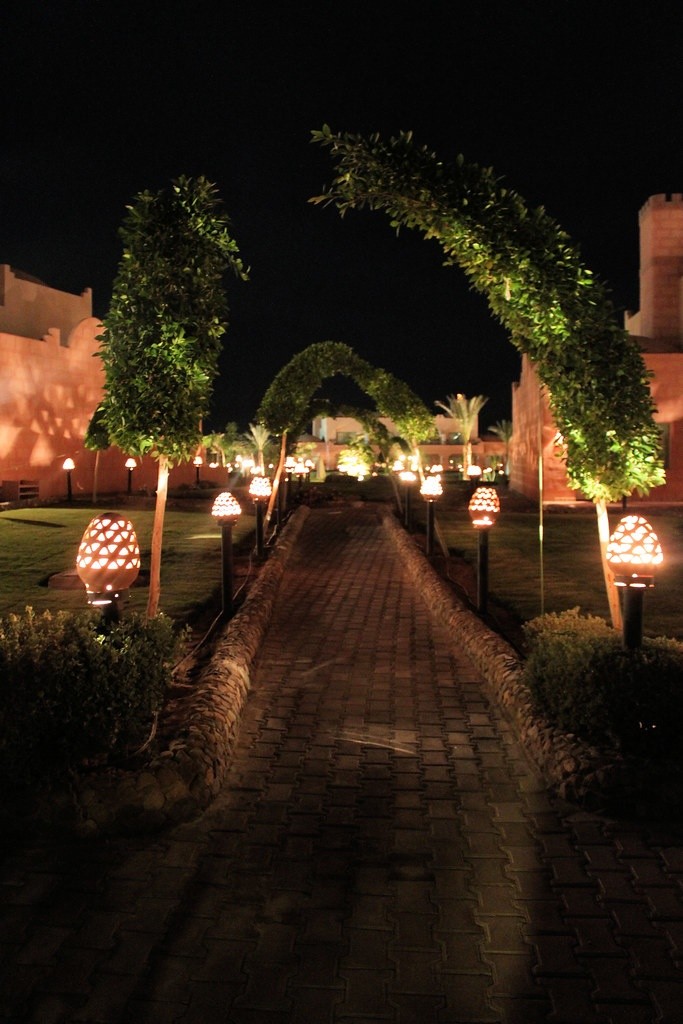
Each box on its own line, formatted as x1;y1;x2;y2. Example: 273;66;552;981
0;479;40;503
